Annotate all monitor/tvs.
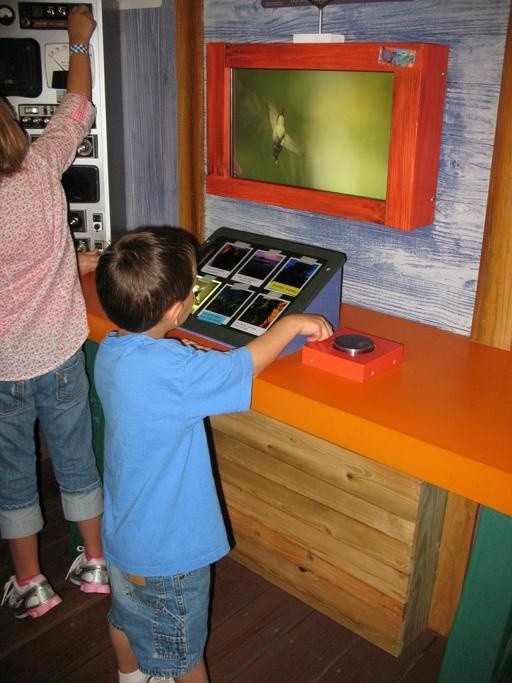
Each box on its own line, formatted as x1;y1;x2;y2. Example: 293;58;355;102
175;225;347;360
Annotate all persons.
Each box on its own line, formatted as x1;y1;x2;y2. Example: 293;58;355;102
0;3;111;620
94;225;335;683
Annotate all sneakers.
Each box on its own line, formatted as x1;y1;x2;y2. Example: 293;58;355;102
141;670;175;683
67;550;111;596
6;570;64;621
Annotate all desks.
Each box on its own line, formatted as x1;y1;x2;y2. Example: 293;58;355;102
68;257;511;683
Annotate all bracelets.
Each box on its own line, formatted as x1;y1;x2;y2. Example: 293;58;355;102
69;45;90;54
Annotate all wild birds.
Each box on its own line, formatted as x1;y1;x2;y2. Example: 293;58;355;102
267;102;304;164
